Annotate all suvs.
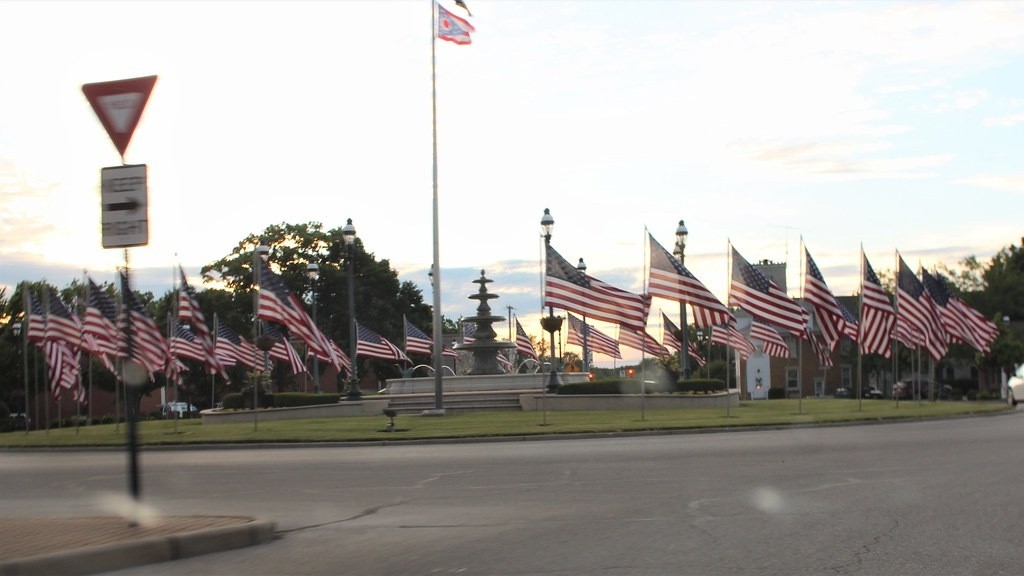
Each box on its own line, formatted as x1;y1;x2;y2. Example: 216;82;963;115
892;379;951;400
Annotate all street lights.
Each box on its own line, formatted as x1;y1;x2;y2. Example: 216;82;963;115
672;220;691;381
576;258;589;371
307;258;322;393
541;208;561;393
451;339;457;375
341;218;364;402
256;242;273;392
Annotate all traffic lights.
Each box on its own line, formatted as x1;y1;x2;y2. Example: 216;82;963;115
629;369;633;374
590;373;593;378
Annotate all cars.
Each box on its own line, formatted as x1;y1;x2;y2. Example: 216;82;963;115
169;402;196;418
865;386;884;398
1007;363;1024;407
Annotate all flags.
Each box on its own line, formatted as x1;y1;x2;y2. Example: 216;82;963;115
804;248;1000;366
647;233;737;329
516;319;541;363
567;312;621;359
406;320;462;360
729;246;809;340
462;316;513;373
750;317;789;358
356;323;414;366
711;325;756;360
545;242;652;327
619;325;669;359
434;1;475;45
662;312;706;367
27;252;357;407
